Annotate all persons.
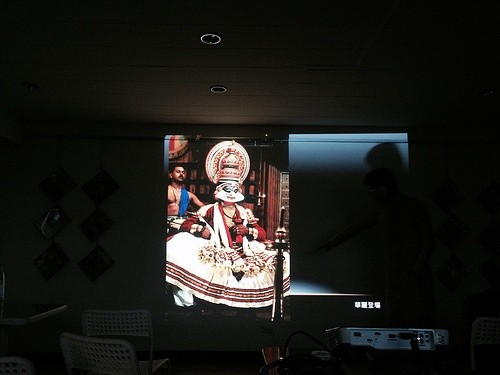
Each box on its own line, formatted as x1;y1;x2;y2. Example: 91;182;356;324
166;179;290;315
168;165;206;219
308;166;434;325
